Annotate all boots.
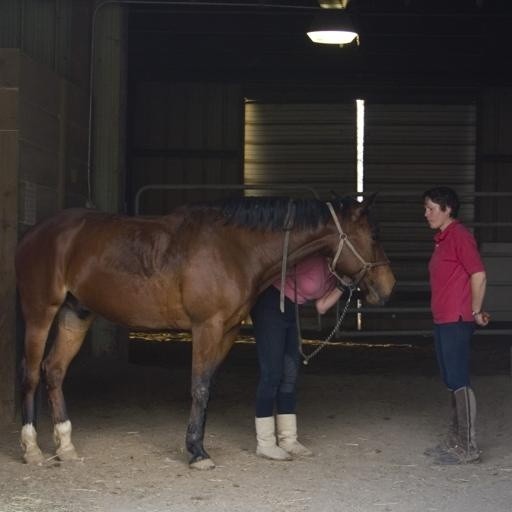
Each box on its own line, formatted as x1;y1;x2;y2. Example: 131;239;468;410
275;413;313;455
424;388;458;455
254;416;293;460
434;387;481;466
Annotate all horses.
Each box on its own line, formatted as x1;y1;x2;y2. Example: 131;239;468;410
15;191;397;470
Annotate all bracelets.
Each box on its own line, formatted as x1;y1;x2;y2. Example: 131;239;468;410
336;283;347;295
470;310;482;318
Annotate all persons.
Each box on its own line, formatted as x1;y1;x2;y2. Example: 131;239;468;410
249;251;351;460
421;186;491;468
